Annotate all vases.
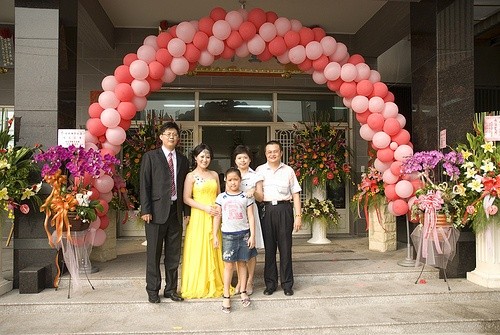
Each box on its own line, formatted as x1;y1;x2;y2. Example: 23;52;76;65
367;196;397;252
55;211;90;232
306;180;332;245
466;220;500;288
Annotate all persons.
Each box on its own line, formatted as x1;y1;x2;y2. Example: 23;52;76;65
224;145;264;296
177;143;234;300
140;122;191;303
213;167;255;313
255;141;302;296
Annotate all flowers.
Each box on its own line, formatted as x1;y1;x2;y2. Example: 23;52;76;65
288;108;359;196
295;196;343;228
122;109;182;229
0;116;46;248
33;145;122;224
400;110;500;229
349;159;395;235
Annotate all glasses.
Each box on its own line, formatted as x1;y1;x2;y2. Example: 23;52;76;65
163;133;179;137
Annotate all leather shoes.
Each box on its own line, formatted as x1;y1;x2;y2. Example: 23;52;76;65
284;288;294;296
263;287;276;295
164;292;184;301
149;294;160;303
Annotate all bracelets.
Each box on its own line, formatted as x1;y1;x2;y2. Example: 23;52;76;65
295;215;301;216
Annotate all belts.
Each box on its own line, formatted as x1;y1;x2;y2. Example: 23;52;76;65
170;201;177;205
264;201;289;205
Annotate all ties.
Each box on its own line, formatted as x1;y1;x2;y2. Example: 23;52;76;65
168;152;176;196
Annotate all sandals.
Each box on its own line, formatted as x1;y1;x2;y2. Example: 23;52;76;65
240;290;251;307
221;297;230;314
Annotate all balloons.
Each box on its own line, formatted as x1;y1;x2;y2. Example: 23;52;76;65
72;7;424;247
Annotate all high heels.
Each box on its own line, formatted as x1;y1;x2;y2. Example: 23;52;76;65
246;281;254;296
234;284;240;294
